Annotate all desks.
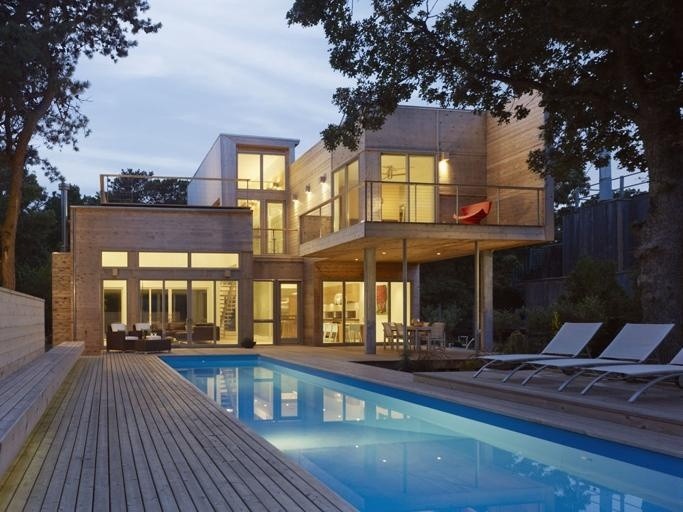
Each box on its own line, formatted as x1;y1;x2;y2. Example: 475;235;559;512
135;339;172;354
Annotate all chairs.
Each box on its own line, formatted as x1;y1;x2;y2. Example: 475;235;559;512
107;323;151;353
166;321;220;345
453;201;492;225
472;321;683;405
382;321;447;352
